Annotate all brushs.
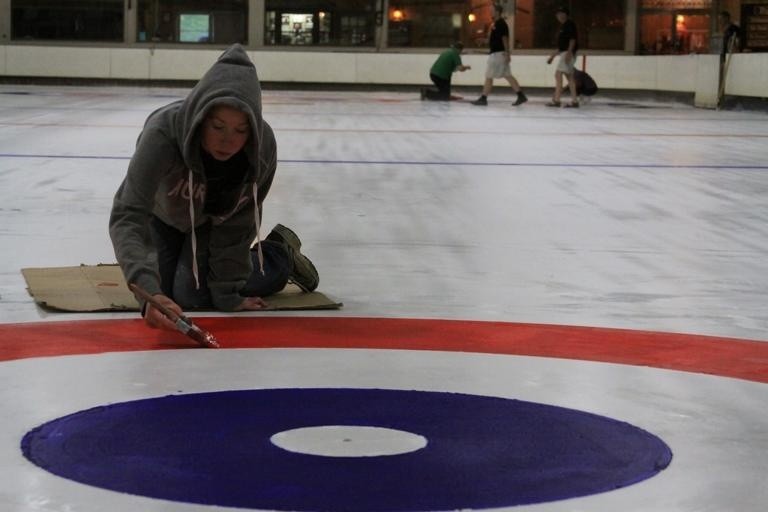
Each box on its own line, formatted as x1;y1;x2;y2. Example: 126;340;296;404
130;284;220;348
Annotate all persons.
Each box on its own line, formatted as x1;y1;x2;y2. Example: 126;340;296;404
420;5;599;108
718;11;741;53
109;43;319;331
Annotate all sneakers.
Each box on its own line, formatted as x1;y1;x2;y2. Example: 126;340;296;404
419;87;428;100
267;224;319;292
544;97;594;108
471;98;489;105
511;94;528;105
